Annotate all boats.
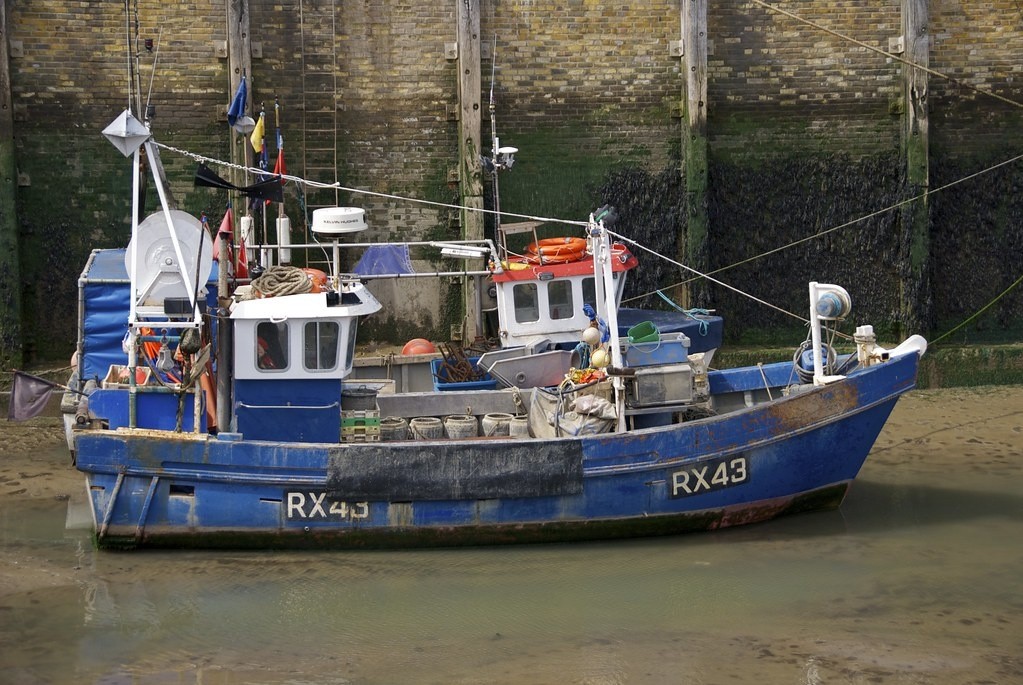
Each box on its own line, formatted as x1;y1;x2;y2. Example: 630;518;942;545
60;102;928;549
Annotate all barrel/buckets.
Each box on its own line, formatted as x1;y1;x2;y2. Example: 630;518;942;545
380;416;410;441
408;416;445;441
627;320;662;353
508;416;530;436
444;413;478;438
342;385;379;412
482;412;516;438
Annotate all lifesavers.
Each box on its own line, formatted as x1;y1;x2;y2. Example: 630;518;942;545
526;250;586;264
294;267;327;286
528;237;587;254
